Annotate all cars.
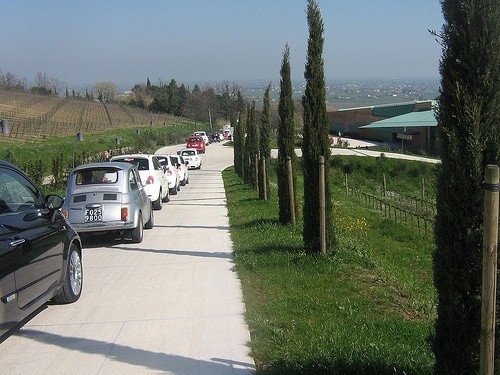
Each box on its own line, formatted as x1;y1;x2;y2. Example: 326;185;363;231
193;130;231;146
0;160;83;337
186;136;206;153
60;162;154;243
102;154;189;210
180;148;202;170
